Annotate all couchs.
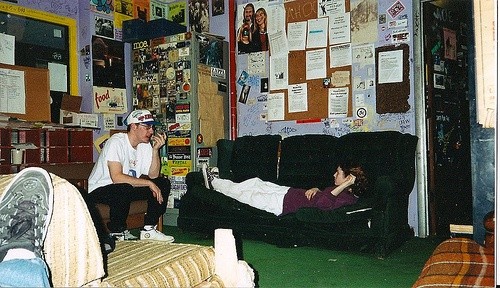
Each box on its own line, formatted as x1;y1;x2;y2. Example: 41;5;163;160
177;131;419;261
411;211;495;288
4;162;163;233
1;173;224;288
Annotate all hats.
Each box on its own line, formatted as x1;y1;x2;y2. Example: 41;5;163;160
123;109;160;126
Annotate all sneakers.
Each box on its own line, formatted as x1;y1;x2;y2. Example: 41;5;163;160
109;229;139;241
202;162;219;189
140;225;175;243
0;166;54;260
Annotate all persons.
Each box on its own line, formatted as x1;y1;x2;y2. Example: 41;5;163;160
189;0;209;33
1;167;56;288
200;161;366;217
240;85;249;103
86;108;176;243
236;3;268;54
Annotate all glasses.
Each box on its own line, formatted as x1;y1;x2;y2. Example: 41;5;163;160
137;124;156;131
